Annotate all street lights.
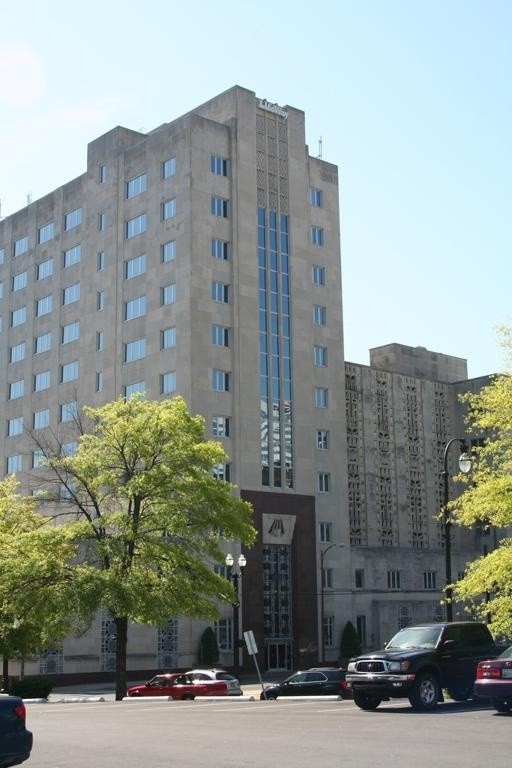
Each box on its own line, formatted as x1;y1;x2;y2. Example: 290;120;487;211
442;438;472;622
319;542;346;666
225;553;247;687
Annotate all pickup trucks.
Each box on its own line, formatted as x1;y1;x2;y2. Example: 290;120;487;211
127;674;227;700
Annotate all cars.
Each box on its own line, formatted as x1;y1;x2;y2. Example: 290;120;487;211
0;696;33;767
185;668;244;696
474;645;512;713
260;667;354;700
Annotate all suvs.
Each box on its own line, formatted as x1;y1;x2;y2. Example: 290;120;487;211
345;621;498;711
